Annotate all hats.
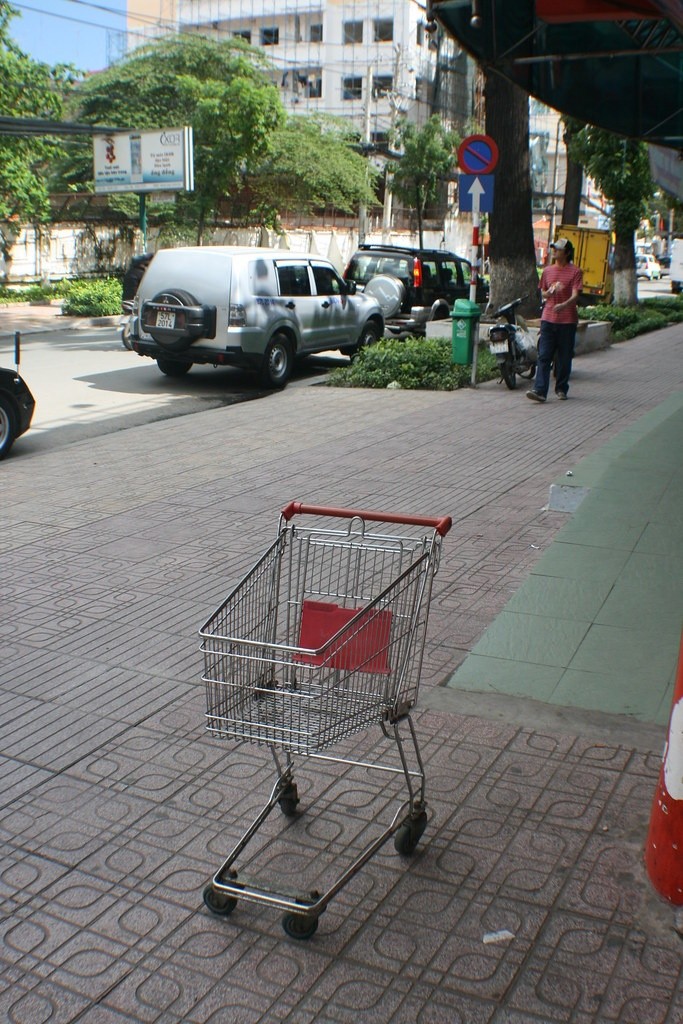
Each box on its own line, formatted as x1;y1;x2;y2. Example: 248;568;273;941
550;238;574;256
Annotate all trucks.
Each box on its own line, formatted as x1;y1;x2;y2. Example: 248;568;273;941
552;224;615;306
670;237;683;294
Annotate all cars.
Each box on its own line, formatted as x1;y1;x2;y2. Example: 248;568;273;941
635;254;660;280
660;256;671;278
0;367;36;459
121;253;156;312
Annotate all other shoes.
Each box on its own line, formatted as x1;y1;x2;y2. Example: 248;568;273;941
526;389;547;403
555;391;568;400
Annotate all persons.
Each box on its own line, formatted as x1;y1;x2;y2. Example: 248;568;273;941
526;238;583;402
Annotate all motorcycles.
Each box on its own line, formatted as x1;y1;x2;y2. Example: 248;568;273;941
485;294;537;390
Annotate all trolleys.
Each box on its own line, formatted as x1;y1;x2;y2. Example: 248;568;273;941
204;501;453;938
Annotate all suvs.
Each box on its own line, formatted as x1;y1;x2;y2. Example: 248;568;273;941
343;243;489;340
130;246;386;390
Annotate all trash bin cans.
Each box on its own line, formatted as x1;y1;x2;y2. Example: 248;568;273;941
449;298;482;366
